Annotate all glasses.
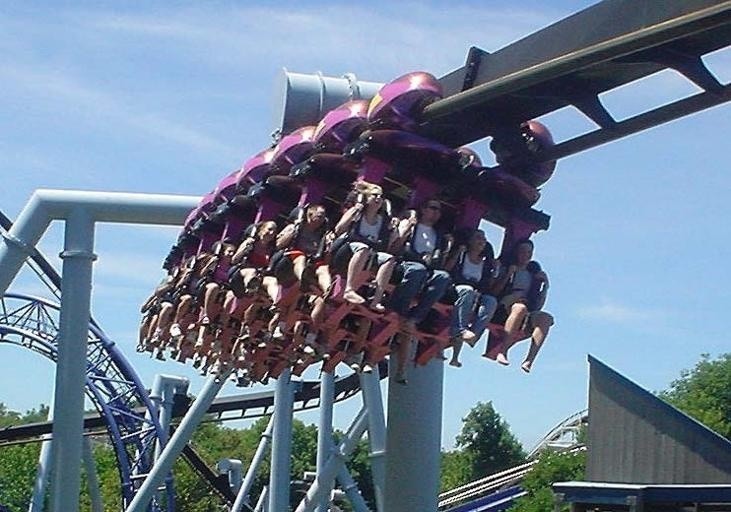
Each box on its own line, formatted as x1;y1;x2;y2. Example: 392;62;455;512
370;193;384;199
424;206;438;210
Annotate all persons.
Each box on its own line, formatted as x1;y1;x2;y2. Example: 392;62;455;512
491;239;555;375
139;182;461;382
448;228;502;348
